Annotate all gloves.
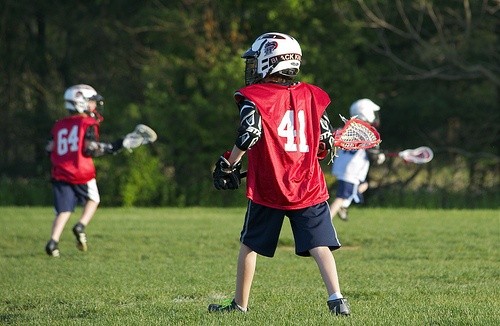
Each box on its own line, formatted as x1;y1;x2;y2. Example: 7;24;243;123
93;142;117;158
212;150;242;190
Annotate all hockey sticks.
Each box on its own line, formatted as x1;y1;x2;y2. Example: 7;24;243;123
240;114;381;179
384;146;434;164
122;124;158;149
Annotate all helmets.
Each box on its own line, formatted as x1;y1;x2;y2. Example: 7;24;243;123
241;32;302;86
350;98;380;123
63;84;104;119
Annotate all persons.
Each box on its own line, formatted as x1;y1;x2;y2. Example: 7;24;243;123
330;99;386;219
209;33;350;314
46;84;143;257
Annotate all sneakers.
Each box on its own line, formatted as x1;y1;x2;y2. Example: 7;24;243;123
72;223;88;252
208;297;249;313
327;298;350;316
45;241;60;257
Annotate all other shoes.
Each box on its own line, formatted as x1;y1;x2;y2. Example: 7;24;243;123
337;208;347;220
